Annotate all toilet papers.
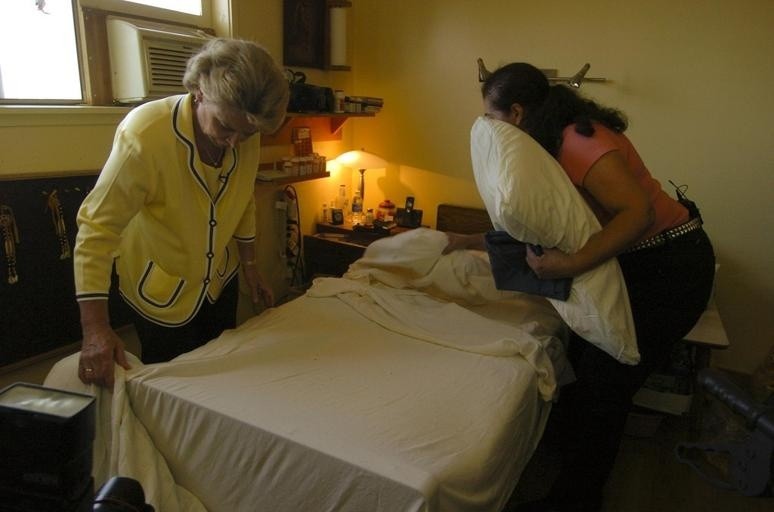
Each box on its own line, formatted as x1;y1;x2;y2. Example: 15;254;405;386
329;8;347;66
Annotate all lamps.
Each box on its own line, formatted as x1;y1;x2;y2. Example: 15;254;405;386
332;149;387;214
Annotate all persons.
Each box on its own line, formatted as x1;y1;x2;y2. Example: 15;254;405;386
72;38;290;394
442;62;716;511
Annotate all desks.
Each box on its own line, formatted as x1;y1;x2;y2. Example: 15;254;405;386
683;293;730;441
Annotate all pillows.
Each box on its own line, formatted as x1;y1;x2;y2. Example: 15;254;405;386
470;117;642;365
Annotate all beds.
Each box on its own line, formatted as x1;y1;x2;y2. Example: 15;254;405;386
118;236;568;512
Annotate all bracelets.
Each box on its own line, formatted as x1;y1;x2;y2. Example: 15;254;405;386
477;232;487;249
240;258;257;266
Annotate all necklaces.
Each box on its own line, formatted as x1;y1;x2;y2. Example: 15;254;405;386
194;115;224;166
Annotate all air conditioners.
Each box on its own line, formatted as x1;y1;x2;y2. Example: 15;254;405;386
105;16;217;106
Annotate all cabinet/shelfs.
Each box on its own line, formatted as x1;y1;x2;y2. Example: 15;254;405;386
255;112;375;188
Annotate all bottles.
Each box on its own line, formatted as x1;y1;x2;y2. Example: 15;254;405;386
284;161;292;176
307;157;313;175
352;190;363;225
299;157;307;177
335;89;345;113
366;209;374;230
321;204;329;224
313;156;320;173
335;185;348;217
320;156;326;172
356;97;362;114
292;158;299;177
348;96;356;113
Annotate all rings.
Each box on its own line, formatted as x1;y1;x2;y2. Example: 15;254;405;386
83;368;93;372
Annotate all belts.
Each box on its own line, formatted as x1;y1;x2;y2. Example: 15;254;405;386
618;215;706;255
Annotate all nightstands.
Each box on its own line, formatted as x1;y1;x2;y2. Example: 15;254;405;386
305;225;414;278
